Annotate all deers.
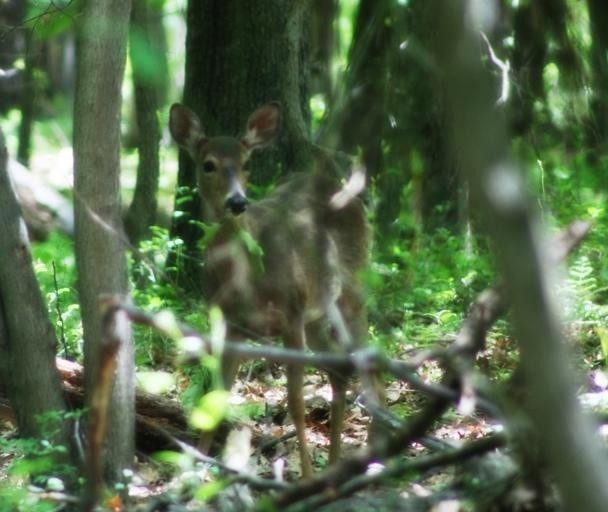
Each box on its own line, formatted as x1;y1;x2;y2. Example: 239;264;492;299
168;100;370;479
